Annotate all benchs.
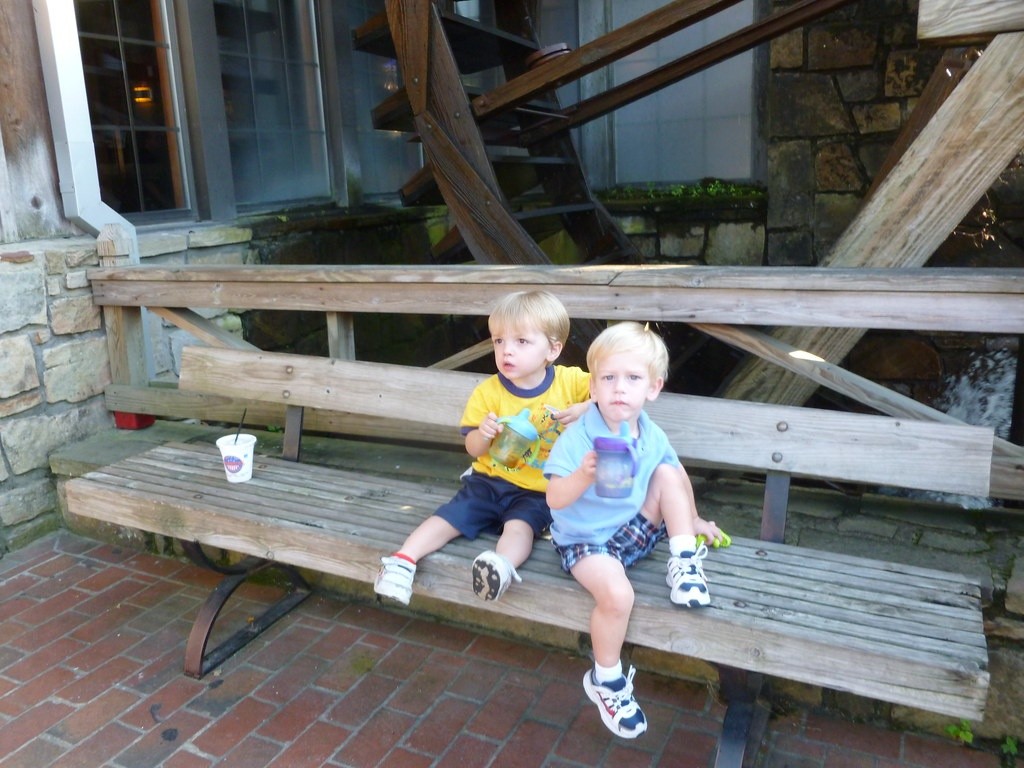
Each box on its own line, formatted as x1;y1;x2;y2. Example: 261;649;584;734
61;347;996;768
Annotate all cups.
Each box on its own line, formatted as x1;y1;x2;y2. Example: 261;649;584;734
216;434;257;484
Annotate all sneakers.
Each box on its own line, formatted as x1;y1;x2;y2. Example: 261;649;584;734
471;549;523;603
373;555;417;607
582;664;648;739
666;540;711;608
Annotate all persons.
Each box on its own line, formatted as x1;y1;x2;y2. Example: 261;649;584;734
546;322;724;739
373;290;591;606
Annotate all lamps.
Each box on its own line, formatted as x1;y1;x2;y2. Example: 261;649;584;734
134;81;152;103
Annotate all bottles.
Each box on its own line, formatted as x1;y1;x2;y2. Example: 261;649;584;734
591;421;639;499
486;409;541;471
